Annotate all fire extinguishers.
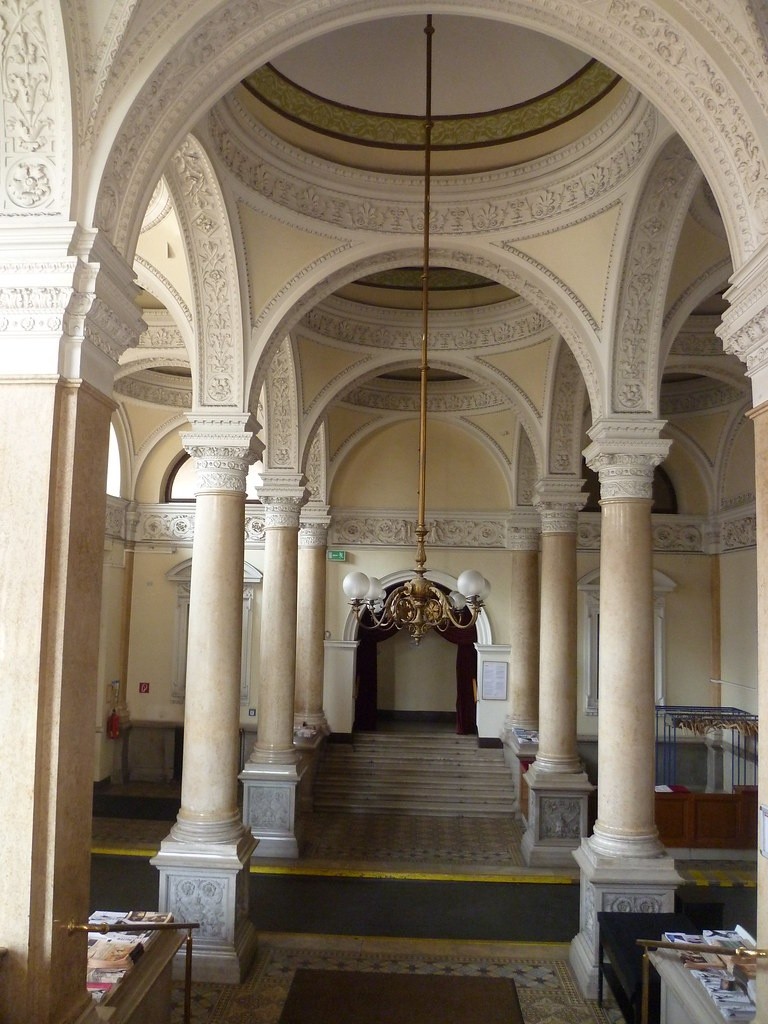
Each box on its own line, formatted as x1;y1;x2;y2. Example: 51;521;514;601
106;707;121;739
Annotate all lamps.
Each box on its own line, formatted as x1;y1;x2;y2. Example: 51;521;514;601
342;12;491;646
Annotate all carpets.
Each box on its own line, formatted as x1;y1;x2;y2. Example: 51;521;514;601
279;967;525;1024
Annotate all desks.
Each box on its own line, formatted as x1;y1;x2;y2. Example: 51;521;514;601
649;935;758;1024
85;910;192;1024
